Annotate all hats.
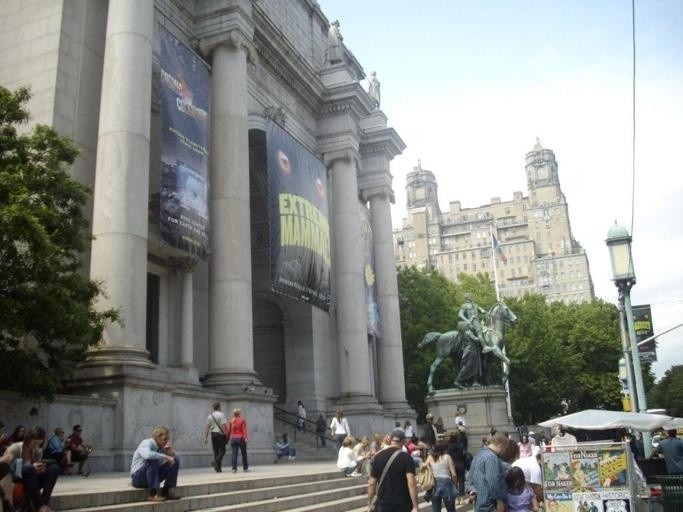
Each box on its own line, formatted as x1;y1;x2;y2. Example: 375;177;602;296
426;414;434;419
391;430;405;442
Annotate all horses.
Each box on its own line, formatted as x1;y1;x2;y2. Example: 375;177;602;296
417;298;518;393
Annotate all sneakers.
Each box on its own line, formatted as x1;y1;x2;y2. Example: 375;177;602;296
148;495;165;501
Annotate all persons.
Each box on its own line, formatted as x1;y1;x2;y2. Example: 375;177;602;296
458;293;492;355
453;320;484;389
205;402;251;472
277;432;296;460
130;427;182;501
316;415;327;446
339;412;683;511
297;401;306;433
330;408;350;452
1;424;93;512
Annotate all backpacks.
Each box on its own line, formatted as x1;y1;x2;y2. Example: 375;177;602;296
419;463;434;490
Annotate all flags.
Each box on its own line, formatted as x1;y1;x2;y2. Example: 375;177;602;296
490;234;507;261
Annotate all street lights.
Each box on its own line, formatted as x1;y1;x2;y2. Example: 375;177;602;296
604;219;655;458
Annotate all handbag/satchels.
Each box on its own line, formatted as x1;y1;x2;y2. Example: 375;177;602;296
370;494;378;511
